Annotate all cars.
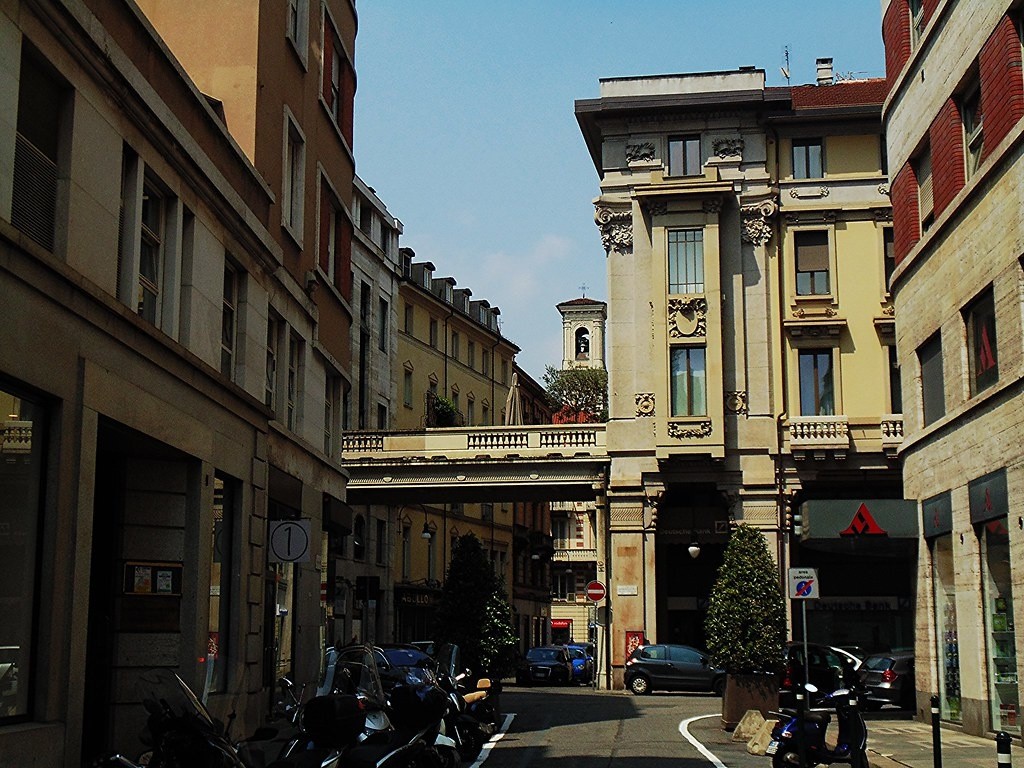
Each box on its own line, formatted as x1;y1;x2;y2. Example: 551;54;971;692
516;646;571;686
0;645;20;698
568;642;597;685
856;651;916;711
778;640;870;705
826;646;868;668
623;644;727;695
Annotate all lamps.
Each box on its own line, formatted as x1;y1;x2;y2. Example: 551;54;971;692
398;499;431;541
518;533;540;558
553;549;572;574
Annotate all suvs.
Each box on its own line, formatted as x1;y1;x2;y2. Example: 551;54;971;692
333;644;440;694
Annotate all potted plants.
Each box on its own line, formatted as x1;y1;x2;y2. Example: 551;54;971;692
699;523;790;731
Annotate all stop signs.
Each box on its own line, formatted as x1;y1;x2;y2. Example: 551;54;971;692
586;581;606;602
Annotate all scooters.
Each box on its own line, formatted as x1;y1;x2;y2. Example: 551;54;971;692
278;641;503;768
768;683;870;768
89;668;247;768
